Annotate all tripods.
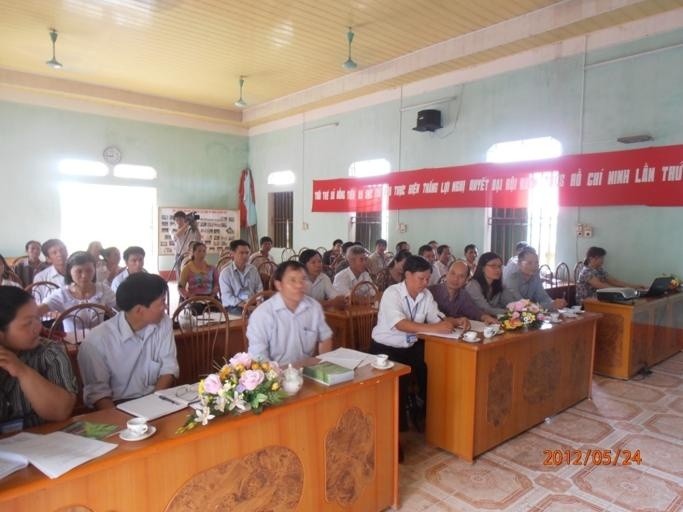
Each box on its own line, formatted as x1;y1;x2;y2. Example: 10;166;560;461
166;228;192;282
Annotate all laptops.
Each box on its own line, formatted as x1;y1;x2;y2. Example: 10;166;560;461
640;277;672;298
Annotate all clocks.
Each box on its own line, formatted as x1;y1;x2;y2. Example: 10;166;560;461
104;145;121;165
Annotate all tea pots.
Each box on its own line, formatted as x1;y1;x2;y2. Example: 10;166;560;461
278;363;306;399
179;313;197;334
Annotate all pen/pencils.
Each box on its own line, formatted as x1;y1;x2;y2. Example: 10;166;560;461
159;395;179;405
195;318;213;320
562;292;566;300
437;316;455;332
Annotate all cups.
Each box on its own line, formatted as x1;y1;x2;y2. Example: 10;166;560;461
547;305;585;320
375;354;389;366
373;300;378;306
483;327;496;338
125;417;150;435
464;332;478;339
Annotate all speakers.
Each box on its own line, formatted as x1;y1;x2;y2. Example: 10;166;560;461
417;109;441;127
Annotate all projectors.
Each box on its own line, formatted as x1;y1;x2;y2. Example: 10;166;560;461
597;287;638;302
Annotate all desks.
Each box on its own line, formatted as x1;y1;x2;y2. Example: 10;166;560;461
579;284;683;381
0;359;413;512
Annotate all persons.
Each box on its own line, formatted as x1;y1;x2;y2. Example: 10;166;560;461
169;212;566;432
1;236;180;430
575;247;646;310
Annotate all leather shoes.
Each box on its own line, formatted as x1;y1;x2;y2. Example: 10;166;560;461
408;393;426;432
398;397;409;432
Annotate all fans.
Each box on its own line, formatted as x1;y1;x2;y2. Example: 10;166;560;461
46;28;62;69
235;75;246;108
340;27;357;69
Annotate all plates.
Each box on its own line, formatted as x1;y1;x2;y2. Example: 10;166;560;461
118;426;157;441
371;306;379;309
372;360;395;370
460;337;481;343
